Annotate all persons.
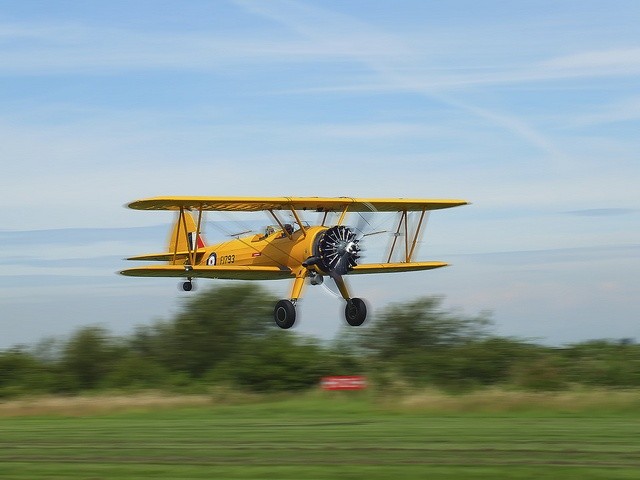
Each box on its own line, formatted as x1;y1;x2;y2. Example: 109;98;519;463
282;223;293;238
265;227;274;237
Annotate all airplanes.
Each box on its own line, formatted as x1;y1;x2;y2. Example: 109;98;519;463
118;196;470;328
